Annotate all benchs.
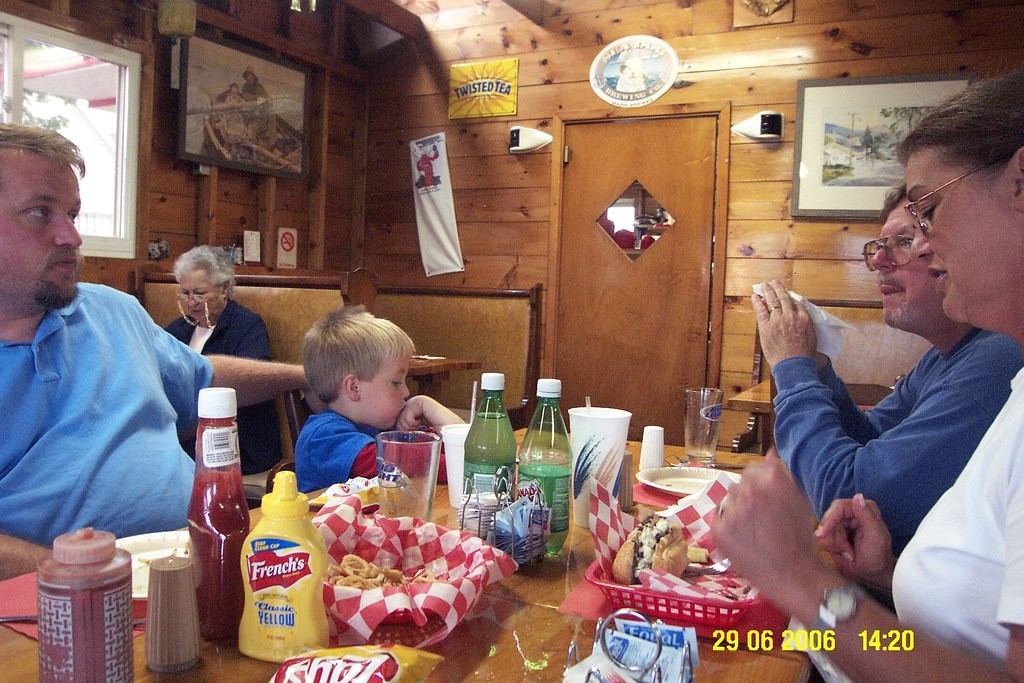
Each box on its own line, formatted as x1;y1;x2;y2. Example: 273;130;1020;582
128;261;382;511
729;297;931;455
368;281;549;431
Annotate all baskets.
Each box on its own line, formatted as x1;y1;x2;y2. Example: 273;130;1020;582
377;608;435;625
584;559;766;629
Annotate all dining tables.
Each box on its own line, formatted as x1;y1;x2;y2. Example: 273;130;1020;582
0;426;817;683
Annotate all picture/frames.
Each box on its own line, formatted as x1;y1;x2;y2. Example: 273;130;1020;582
174;29;310;179
789;70;977;223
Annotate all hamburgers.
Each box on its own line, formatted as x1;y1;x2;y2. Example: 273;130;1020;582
614;515;692;586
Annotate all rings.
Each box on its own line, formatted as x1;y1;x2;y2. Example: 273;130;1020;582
769;306;782;313
718;494;731;515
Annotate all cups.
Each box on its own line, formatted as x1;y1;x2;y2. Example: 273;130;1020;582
373;429;442;521
567;403;631;529
685;385;723;467
638;426;666;469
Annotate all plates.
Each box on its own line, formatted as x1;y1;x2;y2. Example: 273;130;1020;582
637;466;740;500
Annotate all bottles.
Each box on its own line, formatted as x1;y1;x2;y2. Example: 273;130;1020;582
519;376;571;557
463;373;516;497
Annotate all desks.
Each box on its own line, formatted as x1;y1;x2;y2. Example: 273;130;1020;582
729;375;894;452
400;345;483;407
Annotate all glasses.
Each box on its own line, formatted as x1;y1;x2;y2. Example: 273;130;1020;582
861;235;913;272
904;157;1001;239
176;284;226;329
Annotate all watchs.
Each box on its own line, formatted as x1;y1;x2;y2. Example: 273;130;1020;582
809;580;871;645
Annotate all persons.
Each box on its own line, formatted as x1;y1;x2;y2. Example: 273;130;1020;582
751;184;1023;614
293;303;465;494
226;84;246;105
713;67;1024;682
162;246;283;476
615;64;648;93
0;121;329;582
241;64;283;157
